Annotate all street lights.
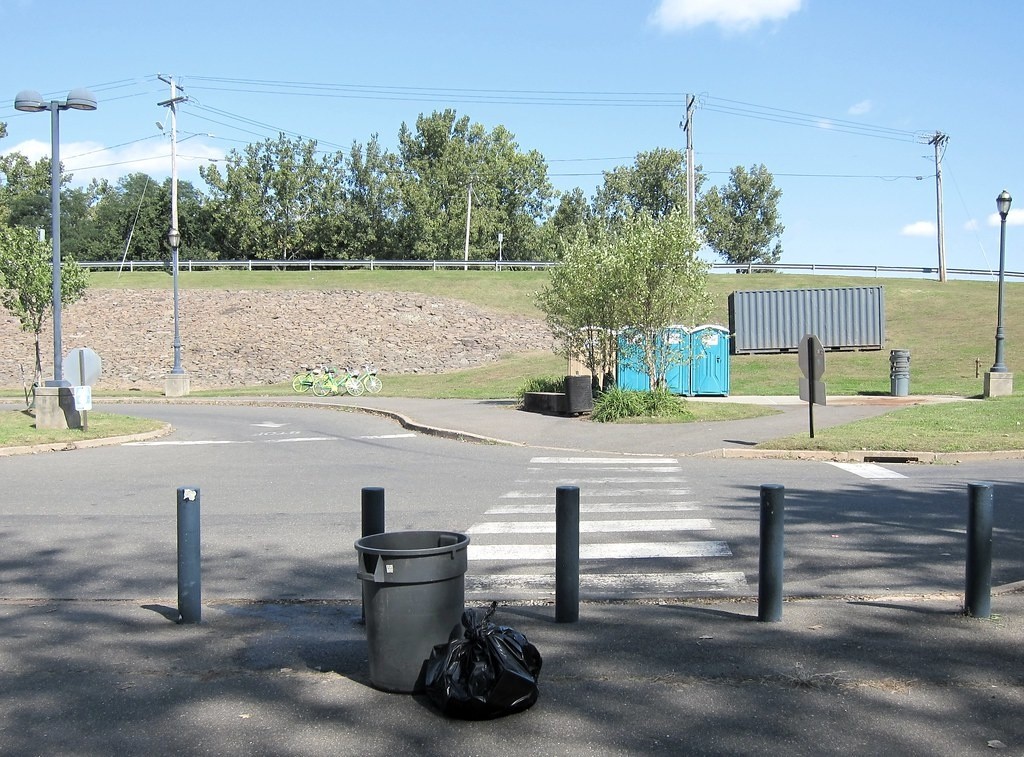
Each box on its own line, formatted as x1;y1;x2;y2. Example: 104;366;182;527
13;87;102;431
984;190;1016;400
154;71;216;398
914;128;950;282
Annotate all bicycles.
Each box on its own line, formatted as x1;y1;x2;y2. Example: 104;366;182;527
291;361;382;397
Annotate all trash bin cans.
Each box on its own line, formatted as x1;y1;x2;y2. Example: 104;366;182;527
355;529;471;690
